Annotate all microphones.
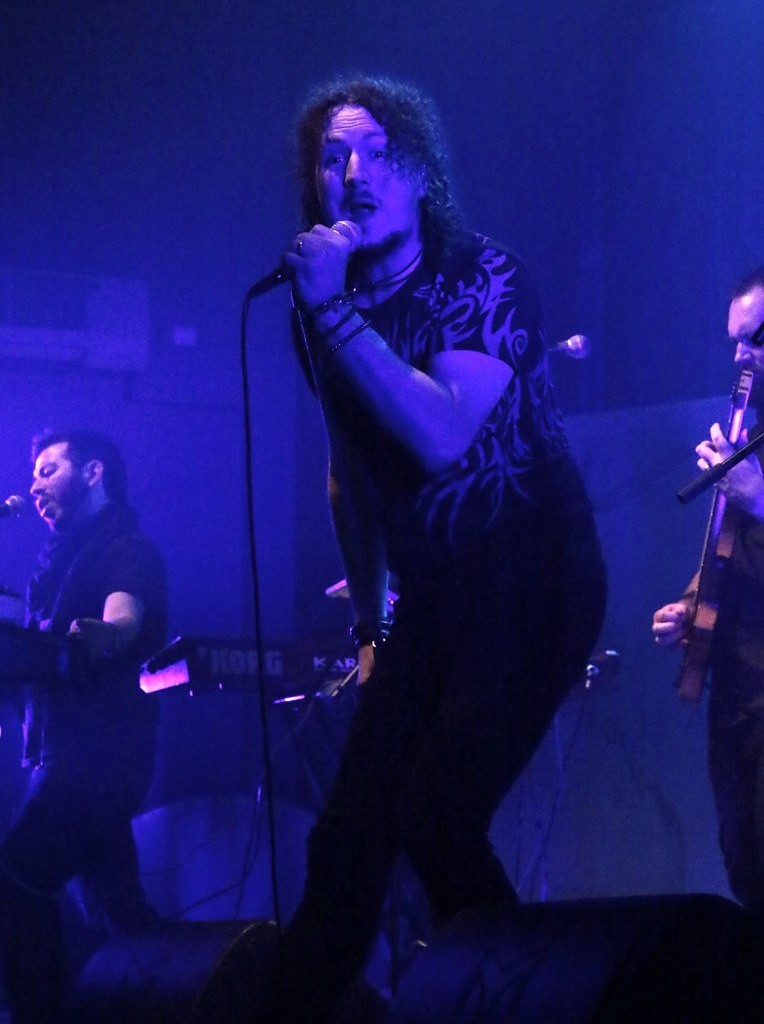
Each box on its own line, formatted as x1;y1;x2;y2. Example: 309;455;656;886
248;219;363;300
547;334;592;370
0;495;26;518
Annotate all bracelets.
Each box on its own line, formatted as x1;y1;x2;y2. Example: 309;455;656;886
311;292;373;358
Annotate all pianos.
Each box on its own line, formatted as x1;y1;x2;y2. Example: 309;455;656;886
137;634;360;699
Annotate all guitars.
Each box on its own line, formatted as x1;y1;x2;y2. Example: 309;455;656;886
666;363;754;707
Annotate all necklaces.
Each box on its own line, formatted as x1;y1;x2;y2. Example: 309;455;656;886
350;248;423;289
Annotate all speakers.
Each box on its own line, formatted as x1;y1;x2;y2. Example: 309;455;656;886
66;919;282;1024
384;892;764;1024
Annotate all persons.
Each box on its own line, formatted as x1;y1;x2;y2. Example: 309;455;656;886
650;268;764;916
0;427;167;1024
249;81;608;1023
